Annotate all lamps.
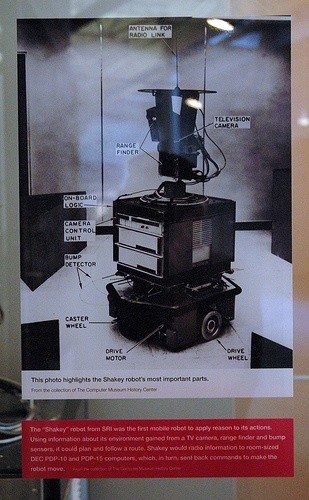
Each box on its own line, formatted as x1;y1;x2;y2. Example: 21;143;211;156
207;18;244;32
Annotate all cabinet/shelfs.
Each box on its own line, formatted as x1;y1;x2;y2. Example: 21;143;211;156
1;378;92;500
